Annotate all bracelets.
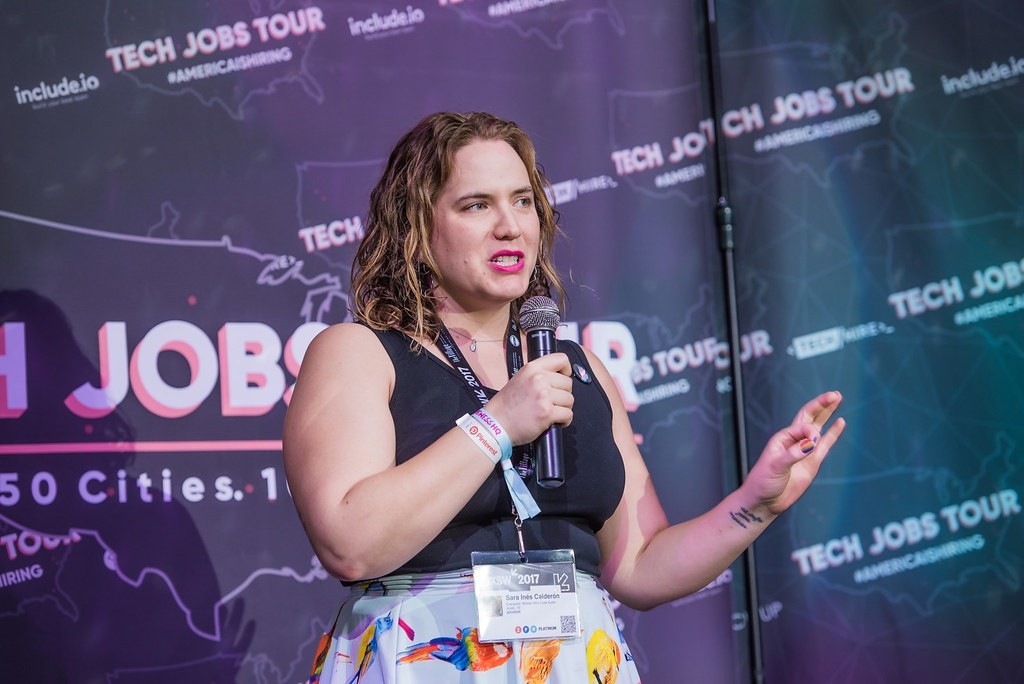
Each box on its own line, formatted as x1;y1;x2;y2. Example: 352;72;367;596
456;407;543;523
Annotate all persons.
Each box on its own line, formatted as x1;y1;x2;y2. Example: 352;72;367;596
283;111;846;683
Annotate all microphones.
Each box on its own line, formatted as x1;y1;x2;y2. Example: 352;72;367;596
519;295;566;489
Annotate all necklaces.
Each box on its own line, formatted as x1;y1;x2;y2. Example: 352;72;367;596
446;326;509;353
